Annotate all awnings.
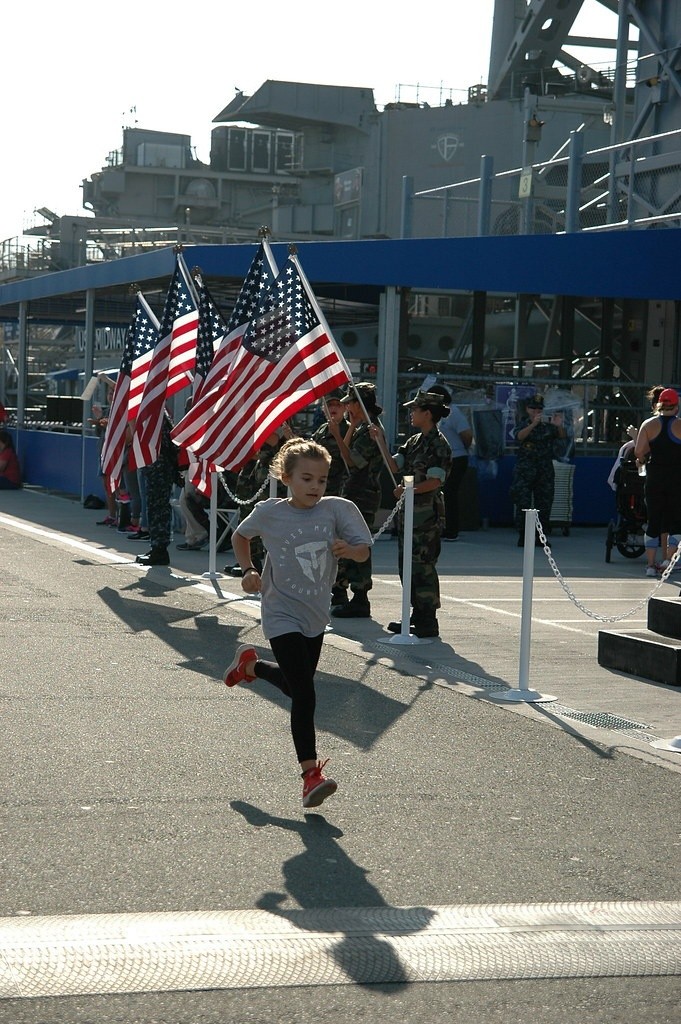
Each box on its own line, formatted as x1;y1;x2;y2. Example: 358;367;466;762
43;367;80;380
99;369;120;377
77;369;102;377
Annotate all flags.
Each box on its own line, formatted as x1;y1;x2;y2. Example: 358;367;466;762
128;253;200;471
176;274;228;498
169;256;353;473
197;243;311;471
100;290;190;496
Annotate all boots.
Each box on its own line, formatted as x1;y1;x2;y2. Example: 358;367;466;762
331;592;371;618
387;608;439;638
331;591;348;605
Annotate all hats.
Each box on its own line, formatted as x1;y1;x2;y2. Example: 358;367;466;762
525;395;545;407
659;388;678;405
324;387;348;405
340;382;376;402
403;391;444;408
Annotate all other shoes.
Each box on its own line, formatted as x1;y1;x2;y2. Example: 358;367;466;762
97;517;265;566
231;561;262;577
535;537;552;548
518;535;526;547
224;562;240;573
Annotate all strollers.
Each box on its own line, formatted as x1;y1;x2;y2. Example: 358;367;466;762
606;441;661;563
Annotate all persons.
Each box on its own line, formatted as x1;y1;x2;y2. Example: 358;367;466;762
429;385;472;541
0;402;21;490
86;370;386;619
628;385;681;576
222;439;373;808
513;396;567;547
368;390;453;638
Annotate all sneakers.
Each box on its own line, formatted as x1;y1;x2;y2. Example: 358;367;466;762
645;565;656;576
656;561;681;572
302;758;338;808
444;533;459;541
223;644;258;688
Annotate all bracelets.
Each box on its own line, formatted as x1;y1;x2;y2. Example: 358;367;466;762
242;567;259;578
